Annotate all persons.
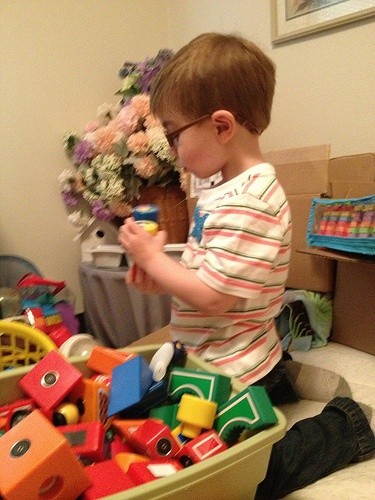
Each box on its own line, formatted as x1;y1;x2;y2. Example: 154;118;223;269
116;31;375;500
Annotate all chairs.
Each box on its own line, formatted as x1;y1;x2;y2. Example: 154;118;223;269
0;255;50;320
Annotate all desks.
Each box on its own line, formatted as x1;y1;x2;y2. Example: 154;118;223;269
79;264;171;349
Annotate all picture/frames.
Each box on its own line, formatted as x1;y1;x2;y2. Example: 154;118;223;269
269;0;375;46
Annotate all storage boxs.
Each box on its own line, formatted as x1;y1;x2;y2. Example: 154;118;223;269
0;343;288;500
266;145;375;355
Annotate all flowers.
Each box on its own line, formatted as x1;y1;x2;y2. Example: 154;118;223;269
59;48;192;227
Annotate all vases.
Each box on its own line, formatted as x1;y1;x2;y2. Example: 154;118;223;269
135;187;188;243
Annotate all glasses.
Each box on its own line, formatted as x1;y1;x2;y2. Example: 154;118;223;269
165;113;213;148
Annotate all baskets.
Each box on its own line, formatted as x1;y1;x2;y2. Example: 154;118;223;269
0;321;59;373
